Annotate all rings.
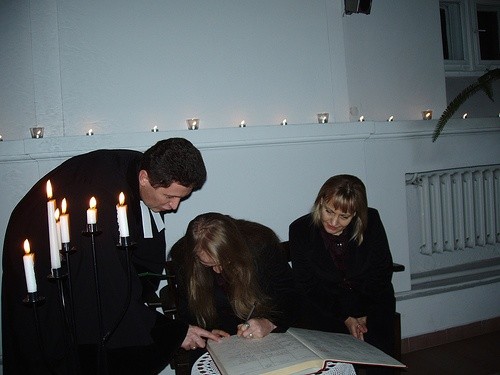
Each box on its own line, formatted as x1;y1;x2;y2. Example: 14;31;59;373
249;333;253;337
192;346;196;349
244;322;250;327
356;322;359;327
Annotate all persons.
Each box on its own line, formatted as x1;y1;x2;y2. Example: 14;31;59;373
166;212;298;338
0;137;207;375
288;174;401;375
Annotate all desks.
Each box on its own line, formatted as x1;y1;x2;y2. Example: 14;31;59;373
189;351;356;375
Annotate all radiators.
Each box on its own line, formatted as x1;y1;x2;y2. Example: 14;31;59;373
407;168;500;255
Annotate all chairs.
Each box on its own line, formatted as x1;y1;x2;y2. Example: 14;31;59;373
283;241;407;360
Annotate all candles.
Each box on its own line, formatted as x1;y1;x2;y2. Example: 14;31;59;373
23;239;38;293
387;115;394;122
86;196;98;224
86;128;94;135
319;116;327;123
357;115;364;121
46;179;62;269
59;198;70;244
424;113;431;119
462;112;469;120
280;119;288;125
191;121;197;129
116;191;130;237
55;207;63;250
151;126;158;132
240;120;247;127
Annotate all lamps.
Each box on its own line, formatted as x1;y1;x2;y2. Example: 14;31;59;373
344;0;373;16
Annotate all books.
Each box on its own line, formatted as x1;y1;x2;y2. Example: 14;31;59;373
206;327;408;375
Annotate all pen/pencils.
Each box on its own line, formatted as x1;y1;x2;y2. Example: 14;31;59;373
238;304;257;339
